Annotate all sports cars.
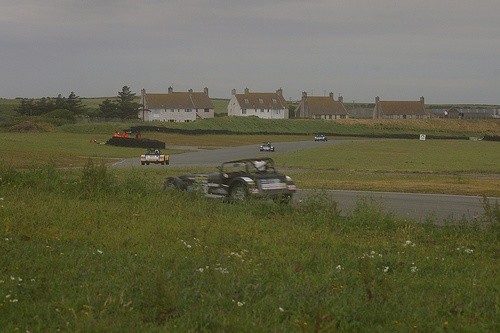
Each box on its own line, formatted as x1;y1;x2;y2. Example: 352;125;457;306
142;148;169;166
163;156;296;207
260;143;274;152
314;133;328;142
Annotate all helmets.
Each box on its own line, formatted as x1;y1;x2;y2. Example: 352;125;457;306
254;160;266;172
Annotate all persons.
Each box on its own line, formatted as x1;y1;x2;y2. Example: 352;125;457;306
114;131;119;136
122;131;128;136
256;160;264;173
